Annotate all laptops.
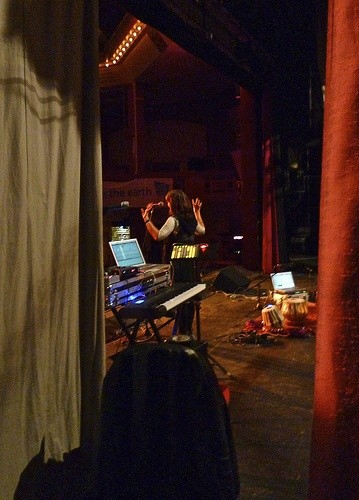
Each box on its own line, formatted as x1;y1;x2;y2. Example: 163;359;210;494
109;238;156;270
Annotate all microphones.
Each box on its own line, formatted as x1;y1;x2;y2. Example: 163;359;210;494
103;202;129;210
153;202;163;207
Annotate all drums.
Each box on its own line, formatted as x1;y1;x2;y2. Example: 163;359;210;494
171;333;199;353
262;305;285;330
281;298;309;325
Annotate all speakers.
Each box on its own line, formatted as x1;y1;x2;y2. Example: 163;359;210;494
212;266;251;294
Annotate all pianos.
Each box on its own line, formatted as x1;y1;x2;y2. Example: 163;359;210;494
118;281;206;320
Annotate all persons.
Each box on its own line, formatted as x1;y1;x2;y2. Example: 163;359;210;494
140;188;205;338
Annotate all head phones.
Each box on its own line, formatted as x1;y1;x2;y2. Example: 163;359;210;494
119;266;139;281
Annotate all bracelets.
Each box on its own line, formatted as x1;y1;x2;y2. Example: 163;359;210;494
144;219;150;224
